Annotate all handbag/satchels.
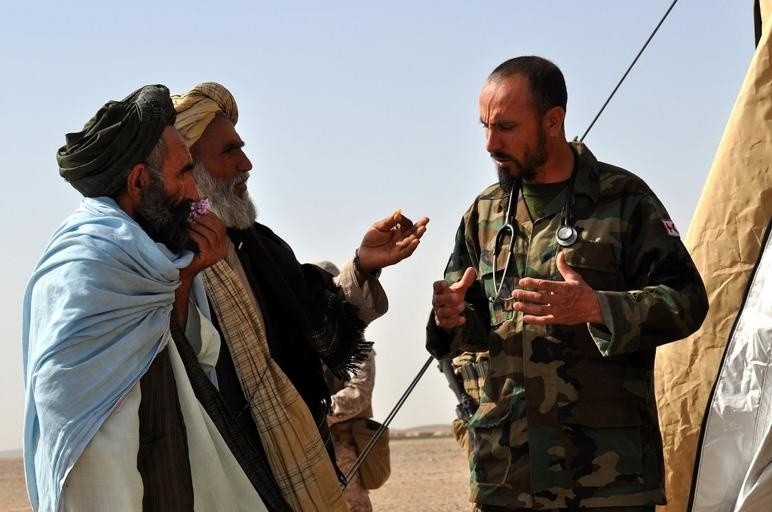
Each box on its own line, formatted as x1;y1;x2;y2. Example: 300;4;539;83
352;420;390;490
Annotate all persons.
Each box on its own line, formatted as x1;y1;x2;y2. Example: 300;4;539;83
170;81;430;511
424;57;711;511
16;86;294;510
312;260;377;512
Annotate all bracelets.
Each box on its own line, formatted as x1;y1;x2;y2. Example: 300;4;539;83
352;249;382;281
